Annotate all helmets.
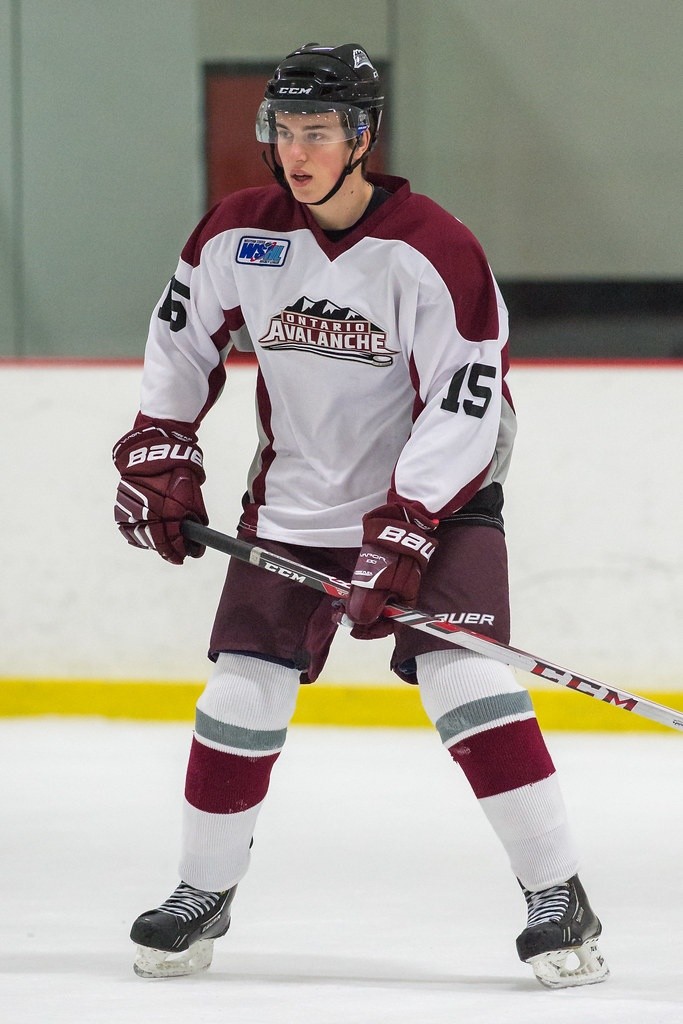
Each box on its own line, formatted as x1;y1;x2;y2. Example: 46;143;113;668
256;42;386;149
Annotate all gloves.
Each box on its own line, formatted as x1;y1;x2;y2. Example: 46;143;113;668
330;488;439;640
113;409;210;565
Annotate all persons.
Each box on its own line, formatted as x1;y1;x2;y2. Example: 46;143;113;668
112;41;611;988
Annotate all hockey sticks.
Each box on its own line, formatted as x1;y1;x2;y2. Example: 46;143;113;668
181;514;682;733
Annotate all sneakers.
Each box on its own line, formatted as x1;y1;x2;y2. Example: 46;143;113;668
129;836;254;978
515;873;610;988
